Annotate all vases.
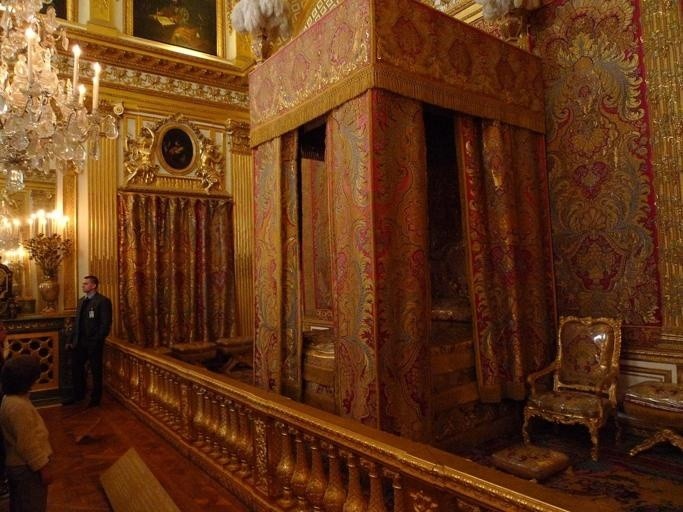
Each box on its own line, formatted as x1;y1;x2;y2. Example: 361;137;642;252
38;276;60;313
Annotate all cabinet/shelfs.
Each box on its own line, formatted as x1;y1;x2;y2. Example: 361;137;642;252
0;314;66;402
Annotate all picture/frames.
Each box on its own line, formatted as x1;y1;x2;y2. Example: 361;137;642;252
156;123;199;176
40;0;79;23
123;0;226;59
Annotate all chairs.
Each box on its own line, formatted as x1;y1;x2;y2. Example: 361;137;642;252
522;316;622;463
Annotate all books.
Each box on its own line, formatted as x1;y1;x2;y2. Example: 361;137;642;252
148;14;176;27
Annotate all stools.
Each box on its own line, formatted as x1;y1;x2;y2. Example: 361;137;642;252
491;445;571;485
156;336;253;375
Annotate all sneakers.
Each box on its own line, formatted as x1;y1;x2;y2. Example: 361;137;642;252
62;396;85;405
87;398;101;406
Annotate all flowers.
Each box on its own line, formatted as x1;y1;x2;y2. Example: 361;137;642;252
24;233;73;277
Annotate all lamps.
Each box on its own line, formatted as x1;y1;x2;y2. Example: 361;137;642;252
0;0;116;195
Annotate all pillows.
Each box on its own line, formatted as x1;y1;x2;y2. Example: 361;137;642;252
432;297;472;322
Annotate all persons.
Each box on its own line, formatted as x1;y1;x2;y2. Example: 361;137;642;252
167;140;189;166
153;0;191;46
0;353;53;512
59;274;112;406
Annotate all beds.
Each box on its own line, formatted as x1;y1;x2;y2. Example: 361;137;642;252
249;0;558;444
